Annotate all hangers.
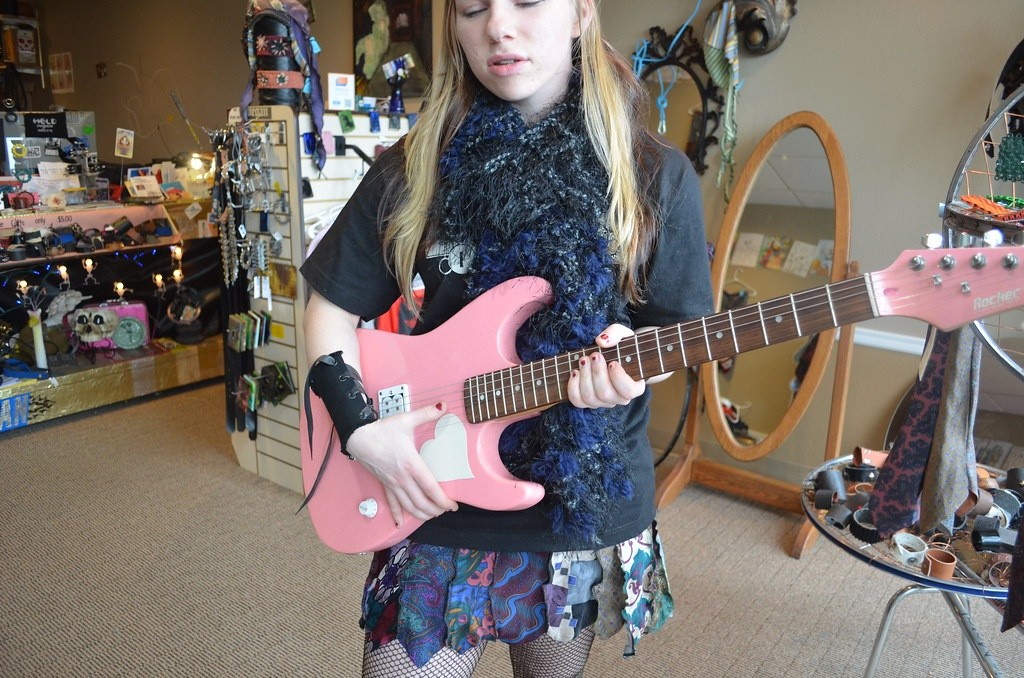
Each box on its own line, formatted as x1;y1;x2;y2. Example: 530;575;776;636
723;268;757;296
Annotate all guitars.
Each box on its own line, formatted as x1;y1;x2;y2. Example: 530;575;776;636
297;245;1024;554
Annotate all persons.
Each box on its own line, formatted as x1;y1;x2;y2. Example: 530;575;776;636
298;0;718;678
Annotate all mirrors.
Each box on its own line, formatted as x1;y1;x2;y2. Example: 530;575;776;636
638;60;708;167
701;111;850;460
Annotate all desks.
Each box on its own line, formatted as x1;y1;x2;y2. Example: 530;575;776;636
800;451;1024;678
